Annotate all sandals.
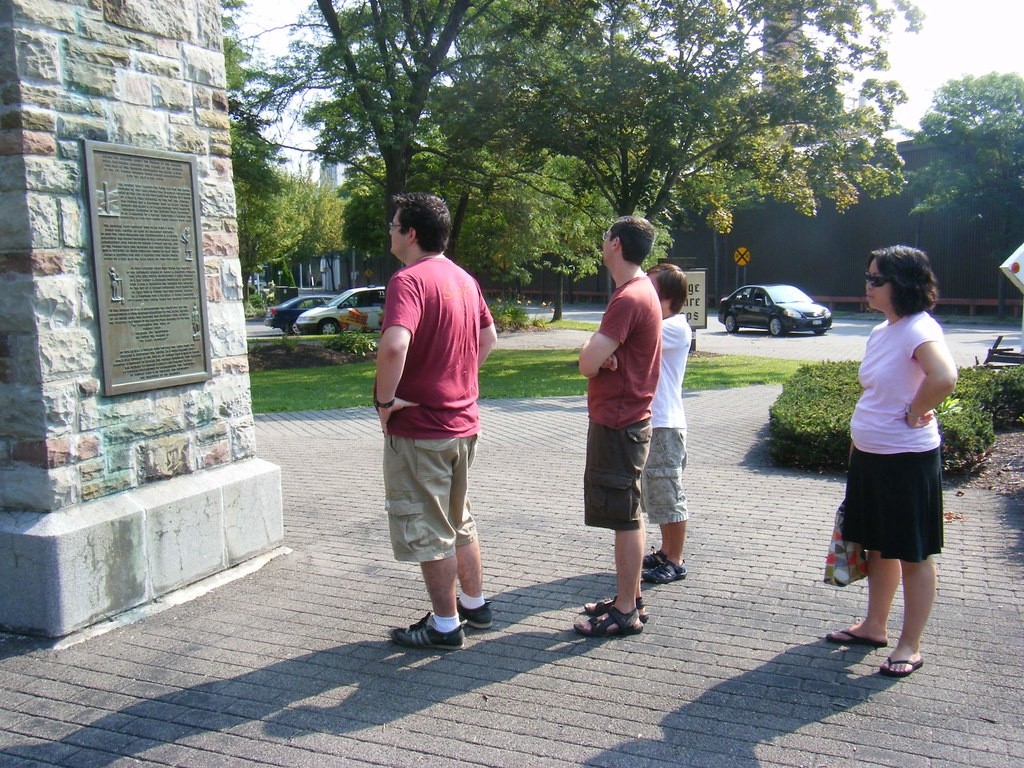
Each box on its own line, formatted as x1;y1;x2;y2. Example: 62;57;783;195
641;550;687;583
573;595;648;637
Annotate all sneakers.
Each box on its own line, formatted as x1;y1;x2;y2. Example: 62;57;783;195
389;612;465;650
456;594;493;629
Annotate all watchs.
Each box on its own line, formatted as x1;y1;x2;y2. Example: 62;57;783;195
374;396;395;408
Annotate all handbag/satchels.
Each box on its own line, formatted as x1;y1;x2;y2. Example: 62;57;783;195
823;500;868;587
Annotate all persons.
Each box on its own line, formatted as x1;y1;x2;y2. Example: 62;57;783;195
373;192;497;650
574;215;691;637
825;245;958;678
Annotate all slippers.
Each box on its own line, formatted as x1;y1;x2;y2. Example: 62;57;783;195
826;628;888;647
880;655;923;678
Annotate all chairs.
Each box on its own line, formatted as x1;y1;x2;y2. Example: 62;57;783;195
741;293;748;300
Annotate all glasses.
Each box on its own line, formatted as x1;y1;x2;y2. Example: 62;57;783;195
865;272;889;287
602;233;611;241
388;222;406;231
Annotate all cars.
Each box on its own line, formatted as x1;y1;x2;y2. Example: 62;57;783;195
718;284;832;337
265;294;341;334
295;285;387;335
